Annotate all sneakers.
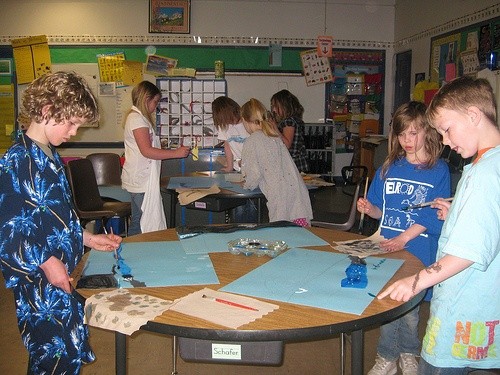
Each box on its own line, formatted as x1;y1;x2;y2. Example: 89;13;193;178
399;354;419;375
366;353;397;375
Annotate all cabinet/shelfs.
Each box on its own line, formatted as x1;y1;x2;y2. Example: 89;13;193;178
155;76;228;149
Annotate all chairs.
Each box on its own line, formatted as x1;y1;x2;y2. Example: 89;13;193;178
64;157;133;237
341;166;372;200
84;153;123;184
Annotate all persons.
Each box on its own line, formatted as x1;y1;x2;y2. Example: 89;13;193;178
0;71;122;375
357;100;450;375
121;80;191;237
378;76;500;374
268;89;310;173
238;98;312;227
212;96;250;173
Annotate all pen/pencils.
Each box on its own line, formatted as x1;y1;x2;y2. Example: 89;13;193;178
197;173;209;176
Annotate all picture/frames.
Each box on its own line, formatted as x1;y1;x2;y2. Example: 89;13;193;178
148;2;190;34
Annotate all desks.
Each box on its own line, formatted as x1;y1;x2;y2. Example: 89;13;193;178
160;169;329;228
68;226;428;374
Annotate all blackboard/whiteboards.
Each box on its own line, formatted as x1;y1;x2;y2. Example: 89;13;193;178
12;63;329;151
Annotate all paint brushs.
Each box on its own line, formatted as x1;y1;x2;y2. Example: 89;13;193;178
180;145;198;160
358;177;369;235
110;227;118;260
202;294;259;312
407;197;453;209
